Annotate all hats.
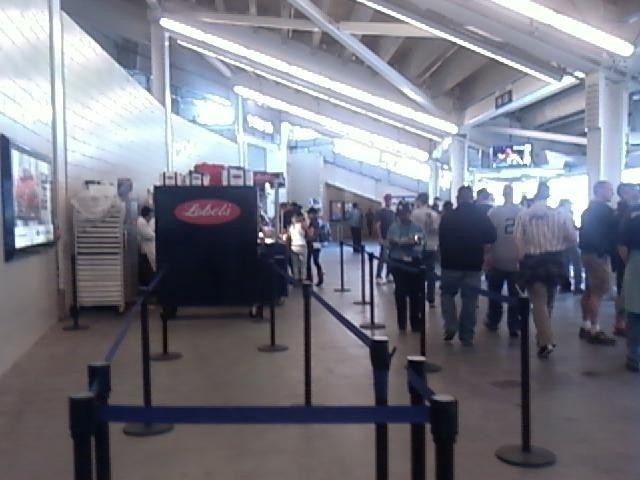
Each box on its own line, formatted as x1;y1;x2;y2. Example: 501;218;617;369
303;208;318;213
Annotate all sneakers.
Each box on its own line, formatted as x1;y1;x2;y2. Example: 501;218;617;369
614;326;627;336
579;327;592;339
409;317;422;333
588;331;616;346
385;274;394;283
484;320;500;332
316;280;324;286
624;363;640;374
461;338;475;346
398;317;408;333
508;329;521;337
443;327;458;341
537;342;556;360
375;277;384;285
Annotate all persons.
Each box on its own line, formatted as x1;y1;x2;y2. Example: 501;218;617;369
142;218;155;277
137;206;155;280
279;201;324;286
376;182;577;358
364;208;374;237
19;169;43;226
347;201;363;253
495;147;513;163
578;180;639;373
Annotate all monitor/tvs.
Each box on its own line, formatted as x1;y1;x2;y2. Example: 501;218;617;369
489;142;533;168
0;133;56;263
